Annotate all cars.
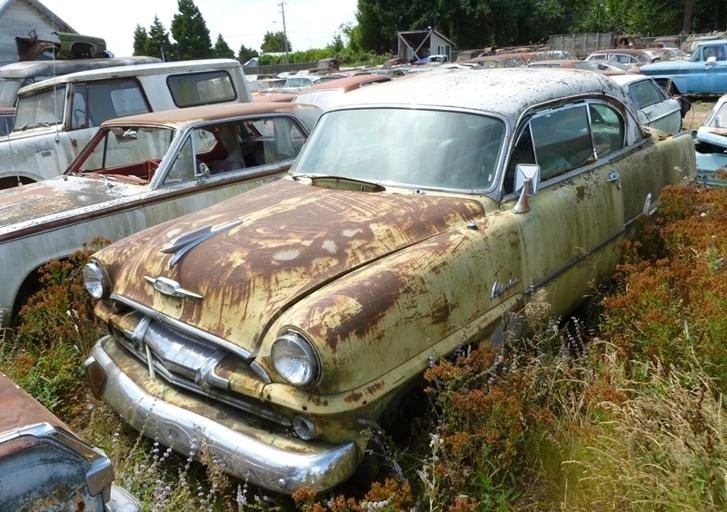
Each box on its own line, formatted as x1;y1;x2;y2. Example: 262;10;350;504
74;70;699;503
0;368;145;512
